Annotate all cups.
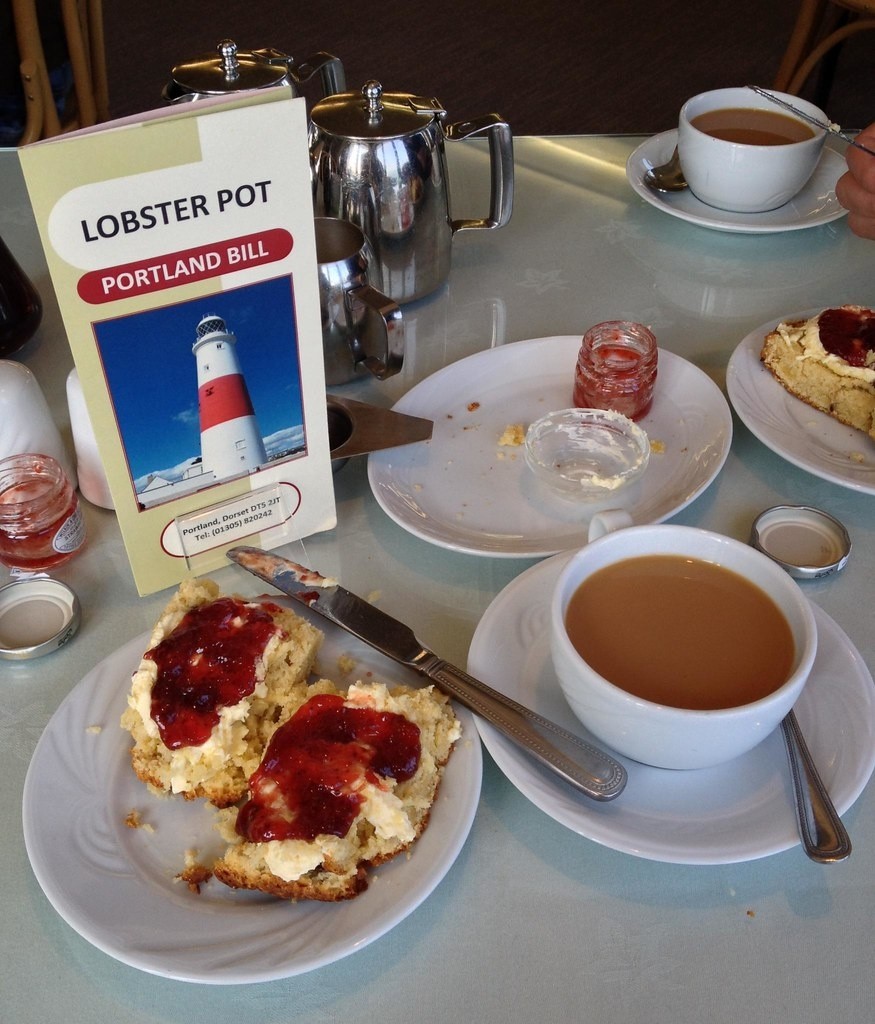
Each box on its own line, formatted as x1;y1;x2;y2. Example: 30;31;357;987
552;510;817;770
680;87;830;212
312;217;405;388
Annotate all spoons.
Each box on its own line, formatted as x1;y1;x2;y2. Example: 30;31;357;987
643;143;687;192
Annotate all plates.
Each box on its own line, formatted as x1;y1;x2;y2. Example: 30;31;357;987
726;306;875;494
627;128;850;232
367;335;733;558
21;597;482;985
468;547;875;867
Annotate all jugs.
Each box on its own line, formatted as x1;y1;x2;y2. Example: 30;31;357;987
165;37;346;98
310;82;516;308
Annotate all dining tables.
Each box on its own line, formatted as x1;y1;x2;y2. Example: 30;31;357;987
1;128;875;1024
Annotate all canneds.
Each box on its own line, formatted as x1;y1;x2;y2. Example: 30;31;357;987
0;453;88;573
572;320;659;422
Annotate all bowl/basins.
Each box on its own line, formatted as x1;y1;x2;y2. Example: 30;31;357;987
525;409;650;503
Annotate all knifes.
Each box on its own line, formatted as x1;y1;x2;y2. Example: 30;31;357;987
226;546;629;801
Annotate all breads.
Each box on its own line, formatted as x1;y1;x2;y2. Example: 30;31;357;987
761;302;875;442
172;677;462;900
115;572;326;807
